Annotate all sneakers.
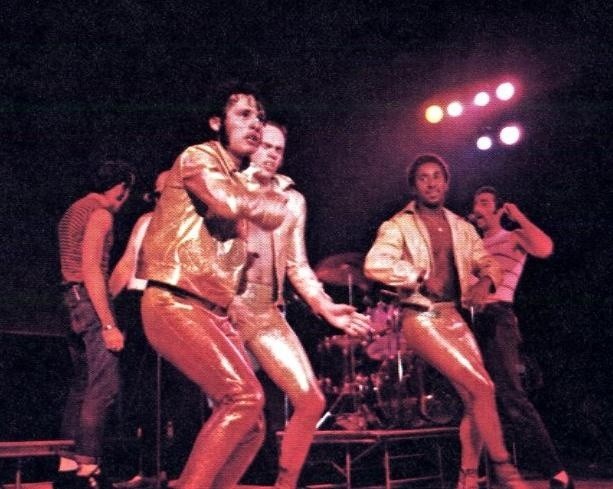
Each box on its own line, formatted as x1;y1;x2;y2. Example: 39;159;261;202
548;478;576;488
52;467;109;488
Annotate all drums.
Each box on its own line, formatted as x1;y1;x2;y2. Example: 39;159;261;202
377;354;461;427
362;307;414;361
316;335;376;394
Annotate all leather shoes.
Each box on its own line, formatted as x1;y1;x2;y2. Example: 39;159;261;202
112;471;167;488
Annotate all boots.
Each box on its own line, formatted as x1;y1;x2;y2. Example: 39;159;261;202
492;461;527;488
457;469;480;489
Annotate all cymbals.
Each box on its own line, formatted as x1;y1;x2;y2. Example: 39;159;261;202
316;253;366;285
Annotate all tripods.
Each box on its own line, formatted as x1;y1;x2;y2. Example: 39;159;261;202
313;343;383;429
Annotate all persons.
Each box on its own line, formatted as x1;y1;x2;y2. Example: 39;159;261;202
53;159;136;488
362;154;533;489
461;185;575;488
135;79;291;488
230;120;376;489
106;168;173;489
